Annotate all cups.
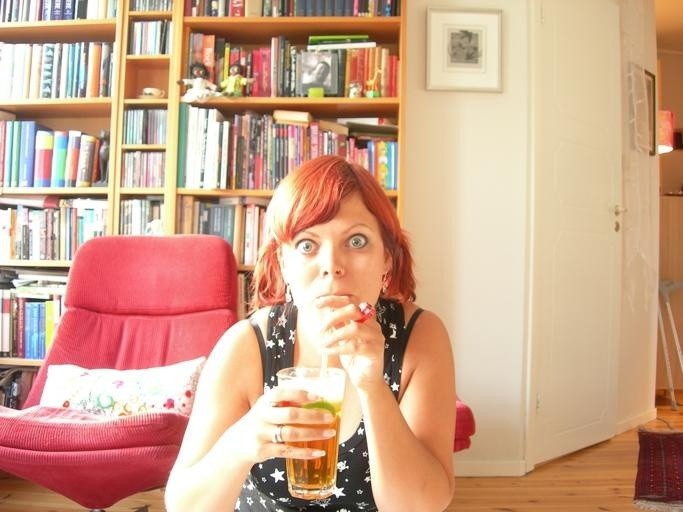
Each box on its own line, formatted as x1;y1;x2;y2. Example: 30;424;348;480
143;88;166;97
276;367;345;500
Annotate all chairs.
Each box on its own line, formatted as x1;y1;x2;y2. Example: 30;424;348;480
0;233;235;511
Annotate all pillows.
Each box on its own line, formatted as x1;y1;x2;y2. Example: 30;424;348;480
38;355;204;416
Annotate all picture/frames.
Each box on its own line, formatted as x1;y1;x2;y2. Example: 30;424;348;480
426;6;504;94
627;61;657;156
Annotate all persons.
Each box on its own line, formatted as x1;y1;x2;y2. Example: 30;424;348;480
164;155;456;512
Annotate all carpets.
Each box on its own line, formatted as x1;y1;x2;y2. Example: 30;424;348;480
635;427;683;512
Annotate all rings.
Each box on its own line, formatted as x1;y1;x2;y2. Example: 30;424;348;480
272;426;284;445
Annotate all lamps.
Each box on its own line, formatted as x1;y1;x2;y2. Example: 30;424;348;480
655;110;676;154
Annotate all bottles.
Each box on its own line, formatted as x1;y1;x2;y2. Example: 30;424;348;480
348;81;363;99
365;81;379;98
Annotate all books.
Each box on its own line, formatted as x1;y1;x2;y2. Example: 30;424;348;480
0;265;68;411
184;1;401;17
176;194;292;321
118;194;164;236
120;107;167;188
180;26;399;97
0;1;117;99
0;110;110;260
127;1;170;56
176;102;398;189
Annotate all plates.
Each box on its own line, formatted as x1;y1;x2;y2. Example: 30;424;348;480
137;95;155;98
